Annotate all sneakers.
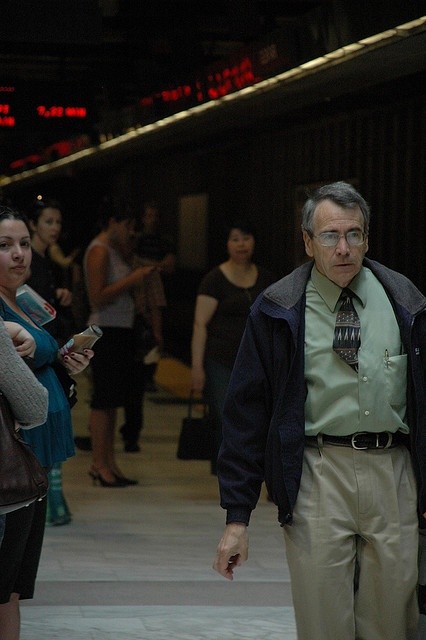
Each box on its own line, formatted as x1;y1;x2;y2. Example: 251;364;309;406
50;490;71;525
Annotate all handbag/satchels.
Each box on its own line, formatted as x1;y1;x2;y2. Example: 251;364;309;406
176;388;217;462
0;392;48;503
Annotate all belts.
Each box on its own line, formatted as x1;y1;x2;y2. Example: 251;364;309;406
320;431;406;450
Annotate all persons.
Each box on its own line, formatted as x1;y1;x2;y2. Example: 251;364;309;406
216;180;426;640
23;194;82;526
2;205;96;474
136;201;179;280
47;225;85;291
2;312;55;640
81;192;156;489
73;215;167;453
190;222;279;477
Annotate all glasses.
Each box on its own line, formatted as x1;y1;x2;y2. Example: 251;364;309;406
302;226;369;247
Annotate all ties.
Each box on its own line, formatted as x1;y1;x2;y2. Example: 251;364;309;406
332;288;360;373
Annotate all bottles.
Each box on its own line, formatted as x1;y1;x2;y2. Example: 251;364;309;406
57;323;105;368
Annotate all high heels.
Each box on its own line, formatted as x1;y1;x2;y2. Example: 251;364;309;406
111;467;138;486
88;462;127;489
